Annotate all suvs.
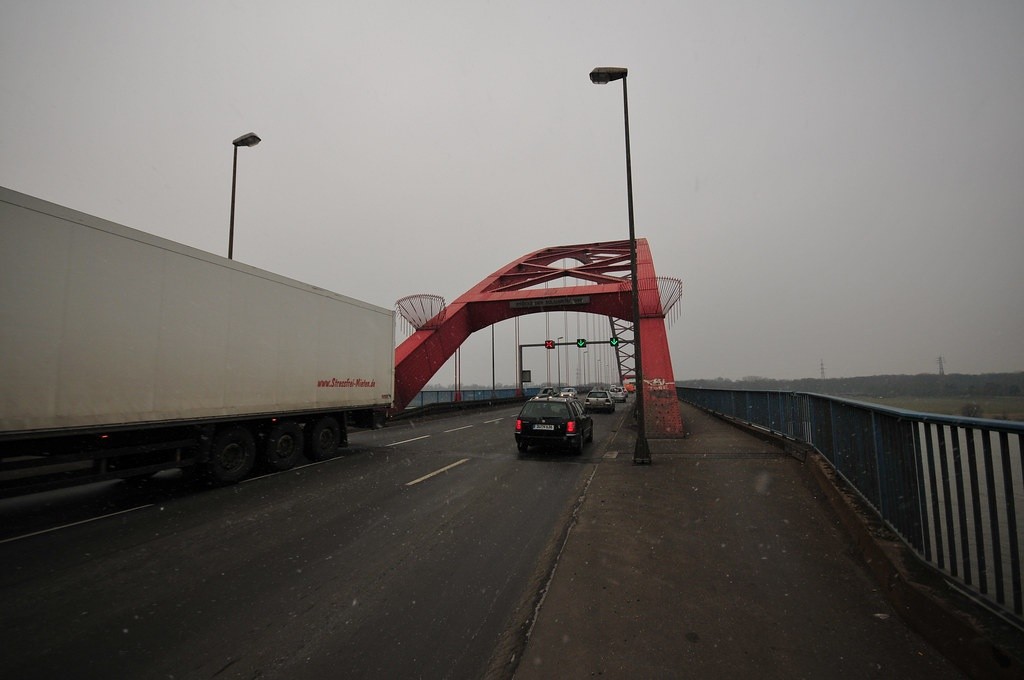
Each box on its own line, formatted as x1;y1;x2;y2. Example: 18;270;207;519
583;390;615;413
515;397;593;454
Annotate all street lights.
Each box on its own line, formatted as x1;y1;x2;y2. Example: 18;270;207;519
228;133;261;259
590;67;653;464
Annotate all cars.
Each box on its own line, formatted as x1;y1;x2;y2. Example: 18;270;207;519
535;387;577;397
593;387;628;402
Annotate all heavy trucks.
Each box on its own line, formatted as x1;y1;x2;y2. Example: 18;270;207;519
0;187;395;488
